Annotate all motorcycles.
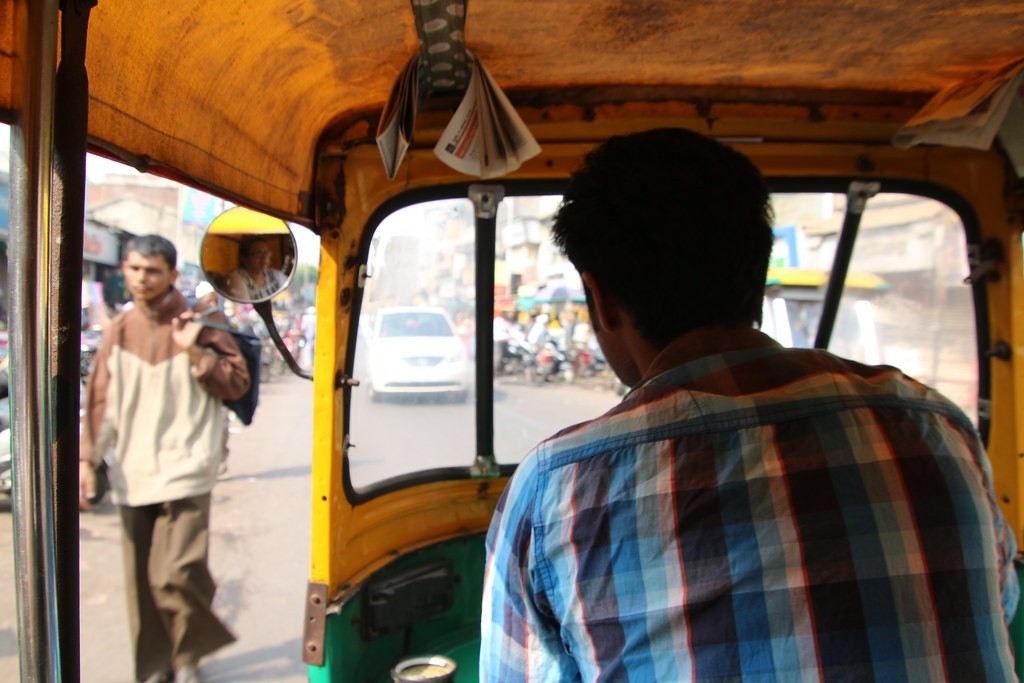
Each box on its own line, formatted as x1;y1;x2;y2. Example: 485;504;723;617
493;312;626;397
0;300;306;513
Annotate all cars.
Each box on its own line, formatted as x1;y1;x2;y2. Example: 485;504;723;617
366;306;469;403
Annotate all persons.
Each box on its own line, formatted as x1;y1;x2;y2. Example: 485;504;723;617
227;235;289;302
478;127;1019;683
79;235;250;683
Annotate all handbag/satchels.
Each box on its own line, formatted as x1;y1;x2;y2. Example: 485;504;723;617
187;307;262;427
